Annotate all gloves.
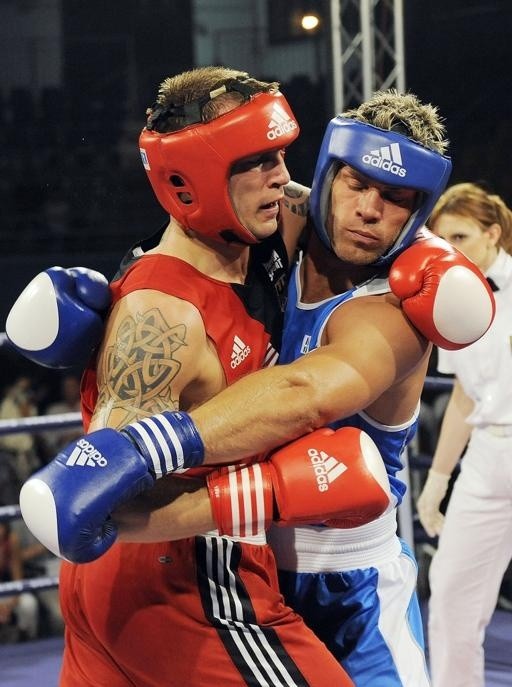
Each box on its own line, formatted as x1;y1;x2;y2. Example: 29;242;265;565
387;228;496;353
17;410;207;566
2;263;115;380
205;426;393;540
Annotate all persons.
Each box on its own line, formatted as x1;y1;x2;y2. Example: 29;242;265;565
0;87;456;687
0;369;511;646
47;62;497;687
411;181;512;687
